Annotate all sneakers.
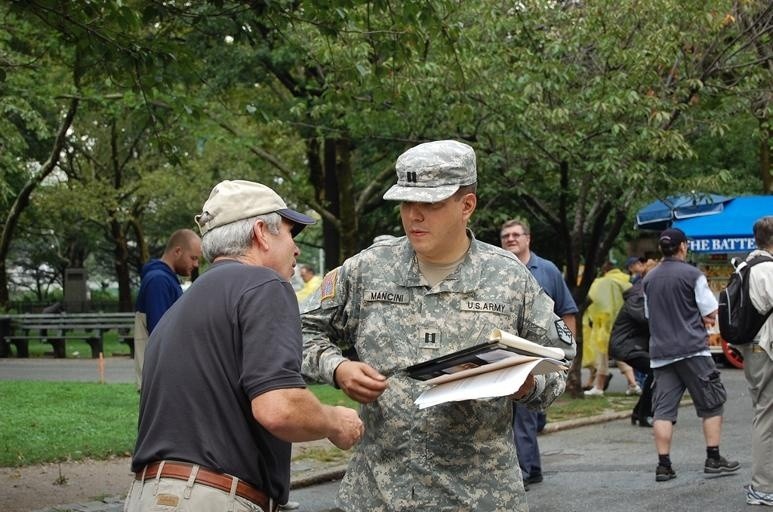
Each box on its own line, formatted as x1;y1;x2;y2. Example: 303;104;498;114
704;456;741;474
280;501;300;510
626;385;642;396
631;414;654;427
656;466;677;481
523;474;542;487
581;373;612;396
744;484;773;507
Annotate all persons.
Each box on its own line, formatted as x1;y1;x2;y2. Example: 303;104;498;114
298;140;579;512
134;228;202;393
500;220;579;491
296;265;323;303
642;228;742;482
581;257;661;427
743;217;773;506
124;179;365;512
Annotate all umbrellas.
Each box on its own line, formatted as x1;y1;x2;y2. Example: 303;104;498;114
635;191;734;231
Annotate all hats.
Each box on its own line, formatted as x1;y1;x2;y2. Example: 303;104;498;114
197;179;317;238
627;256;642;266
383;139;478;205
659;228;689;241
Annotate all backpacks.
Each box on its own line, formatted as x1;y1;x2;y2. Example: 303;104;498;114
718;251;773;345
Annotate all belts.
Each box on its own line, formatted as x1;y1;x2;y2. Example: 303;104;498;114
134;463;279;511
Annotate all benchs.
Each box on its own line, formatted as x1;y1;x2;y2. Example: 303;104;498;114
2;312;135;358
